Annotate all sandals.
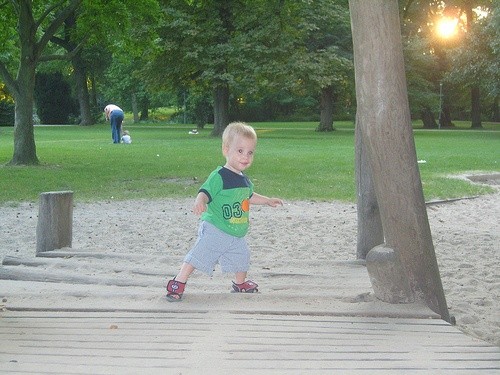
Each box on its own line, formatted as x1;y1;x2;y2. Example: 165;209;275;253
165;276;187;302
230;280;262;294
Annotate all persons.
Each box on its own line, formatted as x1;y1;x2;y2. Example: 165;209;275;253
121;130;132;144
104;104;124;144
166;122;284;301
